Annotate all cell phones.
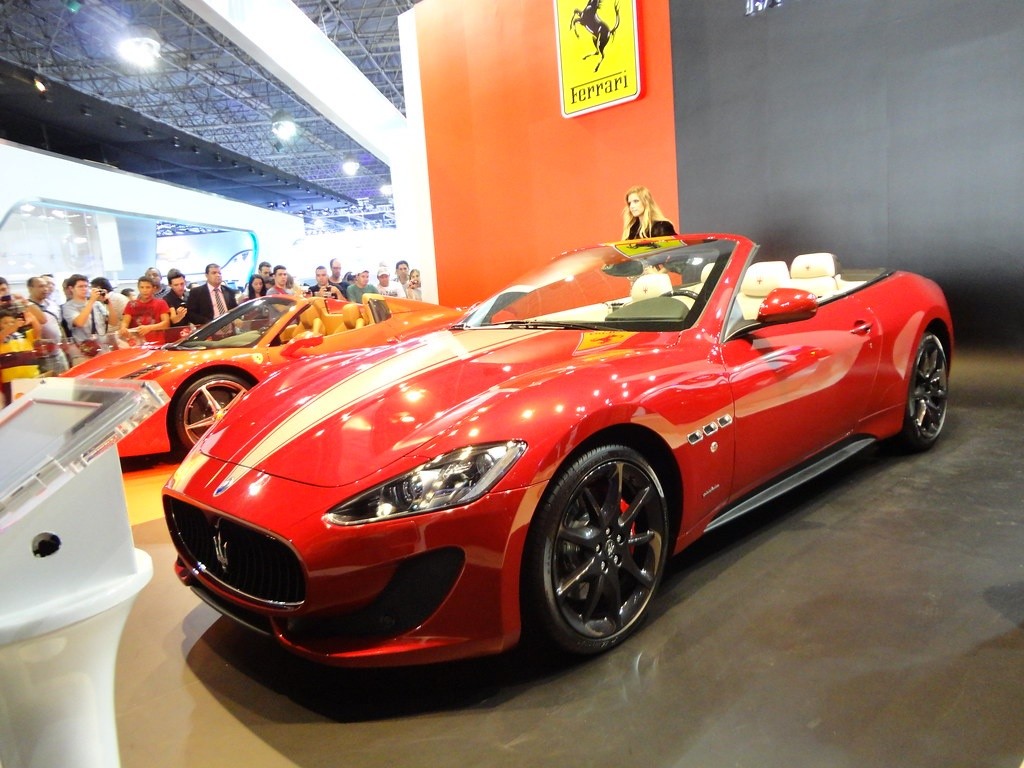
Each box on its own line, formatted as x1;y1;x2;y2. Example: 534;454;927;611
2;295;11;301
326;287;331;291
17;312;25;321
181;302;186;308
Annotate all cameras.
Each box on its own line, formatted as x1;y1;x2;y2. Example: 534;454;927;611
98;289;105;296
411;279;419;284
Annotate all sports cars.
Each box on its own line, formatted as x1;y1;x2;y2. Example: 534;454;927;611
48;294;516;466
158;230;955;672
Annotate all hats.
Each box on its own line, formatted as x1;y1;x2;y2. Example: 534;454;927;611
330;258;342;267
377;267;389;276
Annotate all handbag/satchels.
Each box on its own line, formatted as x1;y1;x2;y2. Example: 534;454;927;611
79;339;101;357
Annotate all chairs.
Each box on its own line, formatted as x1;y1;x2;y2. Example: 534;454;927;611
625;253;870;321
279;293;389;349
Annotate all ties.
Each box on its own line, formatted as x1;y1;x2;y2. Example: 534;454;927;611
213;288;225;315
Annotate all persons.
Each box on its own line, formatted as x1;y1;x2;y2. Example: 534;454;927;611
602;187;685;292
0;257;421;415
